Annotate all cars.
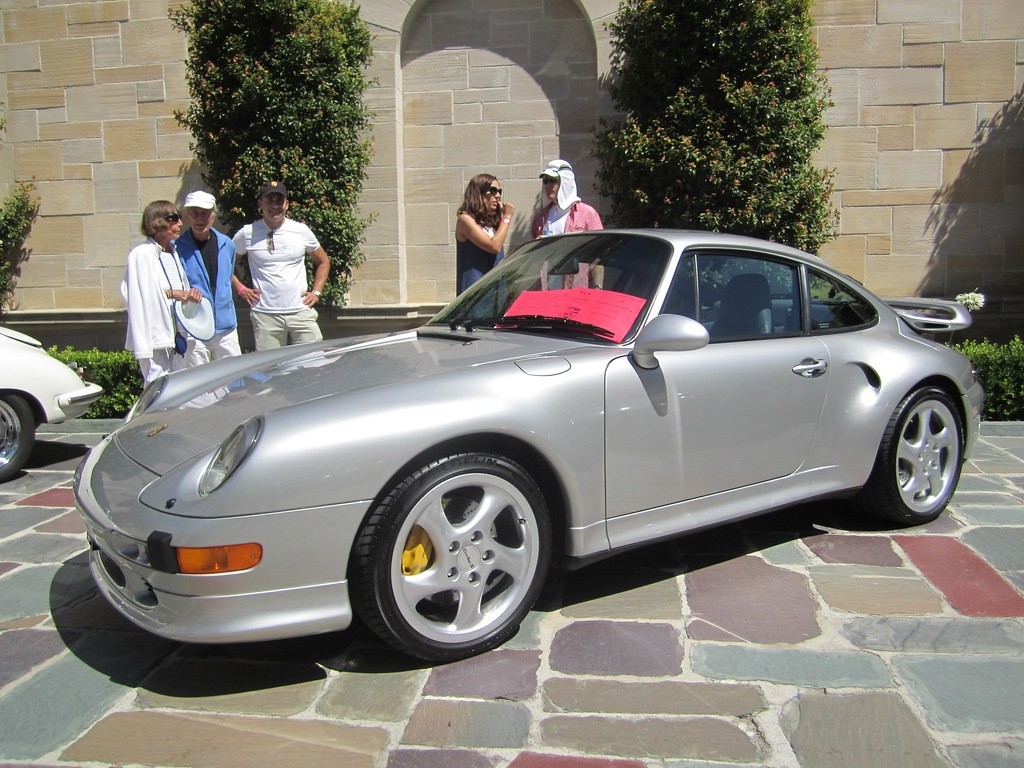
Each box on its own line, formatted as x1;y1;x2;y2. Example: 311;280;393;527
0;326;106;483
74;230;983;660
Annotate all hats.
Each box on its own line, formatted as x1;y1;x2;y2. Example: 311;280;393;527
538;159;573;178
184;190;216;210
173;297;216;341
260;181;286;197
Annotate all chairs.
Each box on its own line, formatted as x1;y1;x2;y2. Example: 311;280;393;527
709;271;774;335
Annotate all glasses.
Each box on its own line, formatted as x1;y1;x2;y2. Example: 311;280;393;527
164;214;182;222
487;186;502;194
543;177;560;185
267;229;274;250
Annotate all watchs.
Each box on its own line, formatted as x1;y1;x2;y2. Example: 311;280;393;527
594;284;603;289
312;290;322;297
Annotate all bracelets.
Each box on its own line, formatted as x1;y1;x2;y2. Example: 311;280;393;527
500;218;511;224
237;286;246;295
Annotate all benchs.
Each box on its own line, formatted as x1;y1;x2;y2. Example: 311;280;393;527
771;299;866;332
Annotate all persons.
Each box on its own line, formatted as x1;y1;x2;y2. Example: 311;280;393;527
531;159;604;291
120;200;203;390
455;174;515;297
169;191;241;369
232;181;330;352
